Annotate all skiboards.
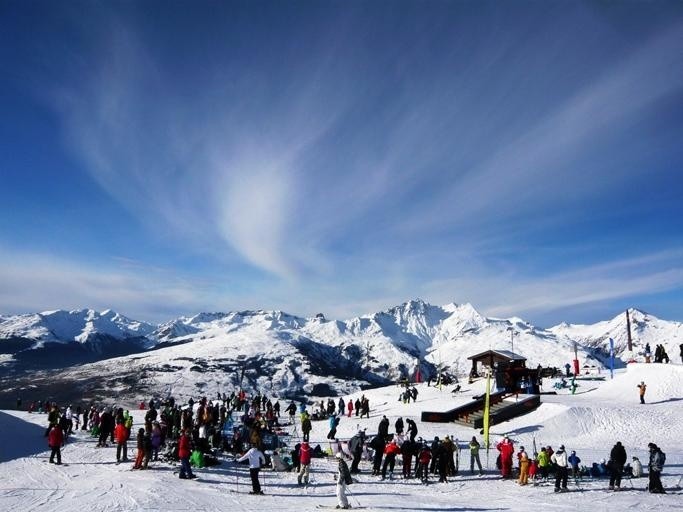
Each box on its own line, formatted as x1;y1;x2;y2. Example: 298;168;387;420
231;489;274;496
129;463;157;470
592;485;683;495
88;443;115;449
289;479;314;490
316;504;369;510
419;478;427;485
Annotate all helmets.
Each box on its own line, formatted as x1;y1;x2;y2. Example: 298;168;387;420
335;452;344;459
360;415;449;448
51;402;73;428
91;405;130;423
303;438;308;444
503;434;576;457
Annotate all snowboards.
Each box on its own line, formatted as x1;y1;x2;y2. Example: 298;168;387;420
42;460;68;466
174;471;200;481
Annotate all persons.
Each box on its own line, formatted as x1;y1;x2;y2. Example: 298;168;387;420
467;435;669;493
270;397;345;485
348;372;458;417
518;358;577;395
332;452;354;509
347;415;457;485
641;341;683;363
135;391;280;491
636;381;646;404
17;398;133;464
